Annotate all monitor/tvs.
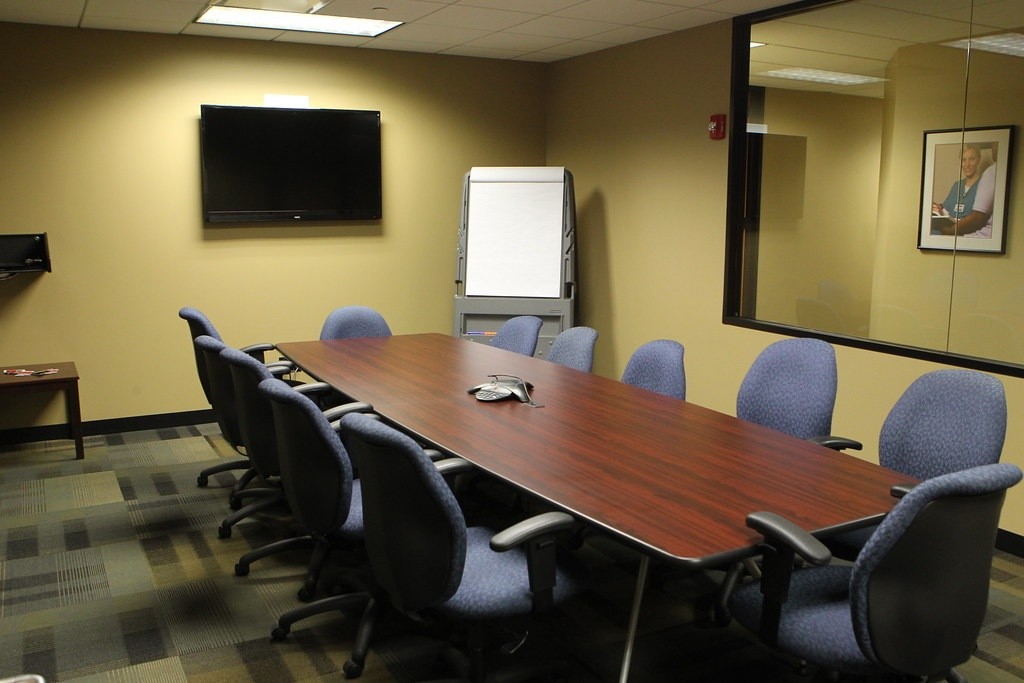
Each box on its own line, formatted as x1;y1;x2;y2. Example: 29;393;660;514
201;104;382;223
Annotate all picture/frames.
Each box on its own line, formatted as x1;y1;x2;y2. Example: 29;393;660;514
917;125;1018;254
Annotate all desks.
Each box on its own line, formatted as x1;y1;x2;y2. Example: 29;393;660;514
275;333;921;683
0;361;85;459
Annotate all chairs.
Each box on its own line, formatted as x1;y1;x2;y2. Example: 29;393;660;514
178;306;373;578
815;368;1007;562
489;316;544;357
711;463;1024;683
256;375;475;679
339;412;593;683
319;304;393;341
544;326;599;375
620;339;686;401
645;337;863;625
